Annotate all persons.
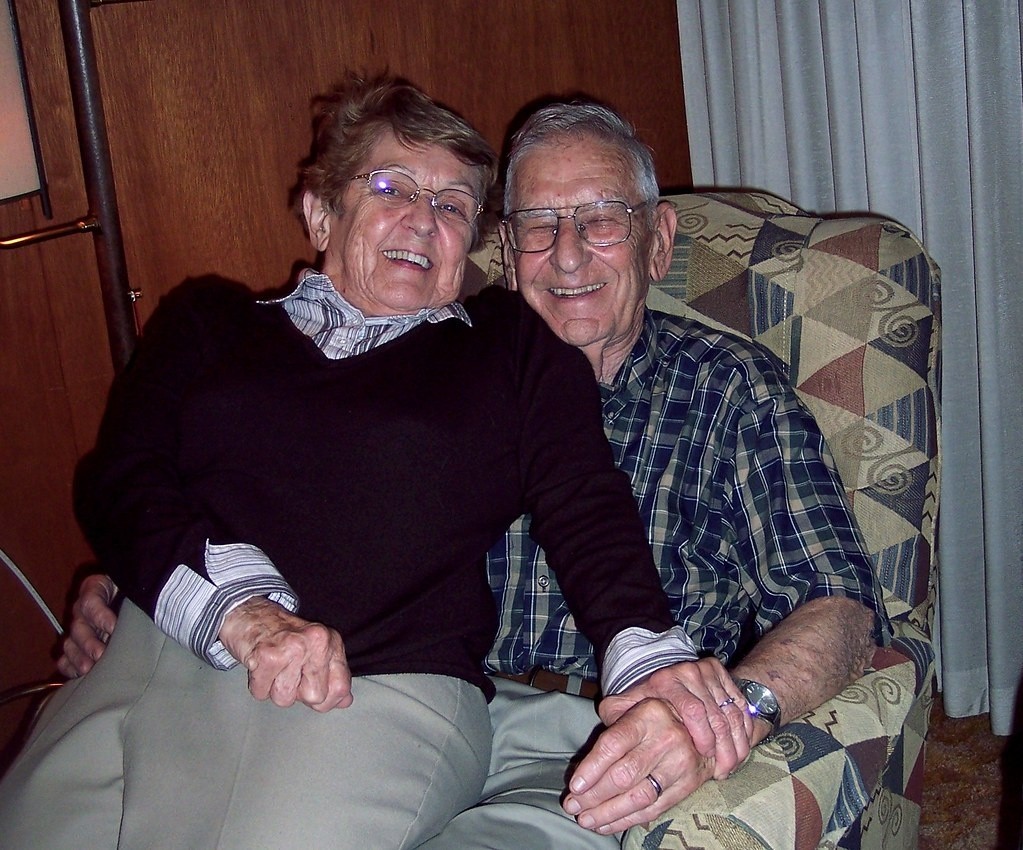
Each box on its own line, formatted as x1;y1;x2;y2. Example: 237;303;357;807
56;99;894;850
1;67;761;850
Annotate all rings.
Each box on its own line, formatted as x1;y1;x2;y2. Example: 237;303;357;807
645;773;662;797
717;696;736;708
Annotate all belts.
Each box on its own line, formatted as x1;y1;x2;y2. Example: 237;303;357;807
486;659;603;700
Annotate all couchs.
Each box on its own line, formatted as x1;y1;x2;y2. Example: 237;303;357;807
438;188;946;850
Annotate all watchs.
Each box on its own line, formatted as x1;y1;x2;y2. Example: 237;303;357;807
728;674;782;746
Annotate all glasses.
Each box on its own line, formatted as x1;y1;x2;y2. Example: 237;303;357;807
497;200;650;253
350;170;483;227
